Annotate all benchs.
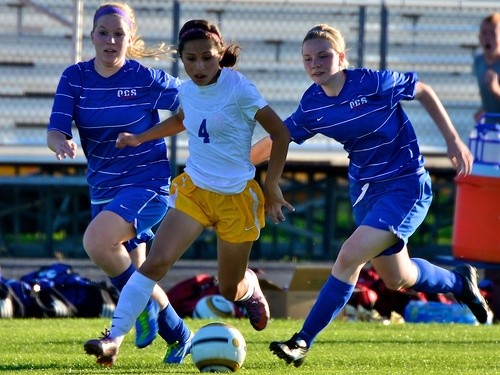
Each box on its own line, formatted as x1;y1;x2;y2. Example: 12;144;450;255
1;1;500;163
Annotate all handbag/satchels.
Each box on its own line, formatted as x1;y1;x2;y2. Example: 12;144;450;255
0;264;119;319
166;274;244;319
345;264;493;319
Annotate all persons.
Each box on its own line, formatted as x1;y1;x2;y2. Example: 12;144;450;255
250;23;487;368
48;2;193;364
471;11;500;122
83;21;296;366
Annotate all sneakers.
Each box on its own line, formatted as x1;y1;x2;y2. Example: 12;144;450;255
135;299;158;349
452;264;488;325
241;268;270;331
269;333;310;368
83;329;118;367
162;327;195;364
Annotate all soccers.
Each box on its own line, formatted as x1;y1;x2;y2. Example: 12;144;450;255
190;322;248;373
192;294;236;320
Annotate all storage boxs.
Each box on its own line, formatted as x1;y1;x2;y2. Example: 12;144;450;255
261;268;334;318
454;165;500;263
